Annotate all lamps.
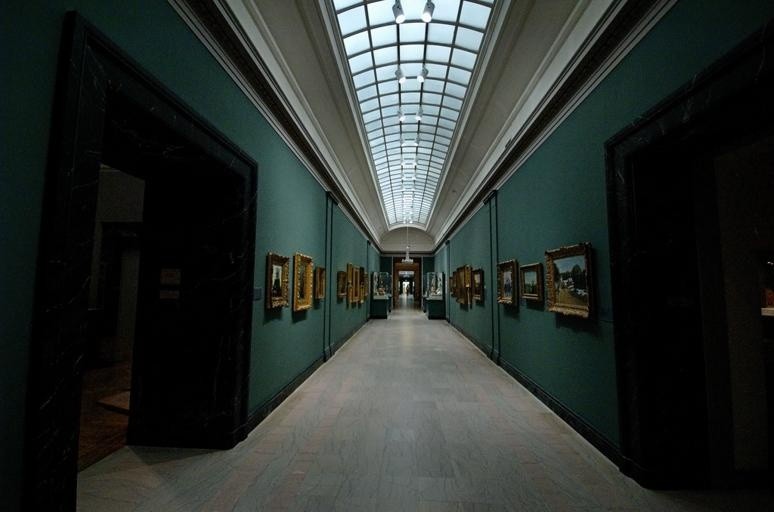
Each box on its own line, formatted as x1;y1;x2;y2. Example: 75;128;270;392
394;67;428;84
396;106;423;121
398;134;420;147
392;0;434;23
401;227;414;266
400;156;418;223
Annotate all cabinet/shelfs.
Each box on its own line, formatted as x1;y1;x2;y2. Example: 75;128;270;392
422;272;445;320
371;272;392;319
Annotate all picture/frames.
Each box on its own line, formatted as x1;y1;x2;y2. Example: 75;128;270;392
448;264;484;306
314;266;326;300
543;240;598;324
292;251;314;313
265;251;290;311
518;262;544;303
496;259;518;308
337;262;369;305
